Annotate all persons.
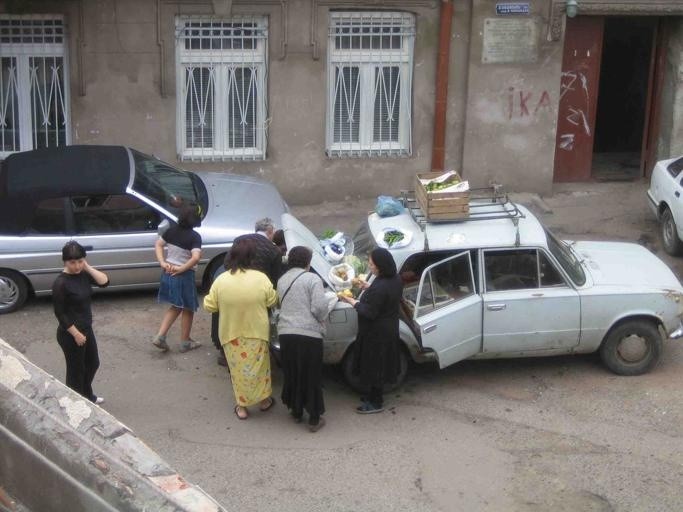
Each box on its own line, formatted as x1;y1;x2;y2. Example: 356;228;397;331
337;246;405;416
195;212;341;435
49;239;111;406
147;200;204;355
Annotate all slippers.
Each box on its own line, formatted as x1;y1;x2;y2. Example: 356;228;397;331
260;396;273;411
235;405;248;420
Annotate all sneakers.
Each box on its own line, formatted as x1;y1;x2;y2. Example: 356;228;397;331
153;336;170;350
308;417;325;432
95;397;104;404
355;395;384;414
180;340;201;353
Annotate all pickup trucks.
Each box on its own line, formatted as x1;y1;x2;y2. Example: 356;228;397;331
648;148;681;253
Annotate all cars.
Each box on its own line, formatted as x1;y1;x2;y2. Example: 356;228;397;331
270;193;682;394
0;146;292;313
